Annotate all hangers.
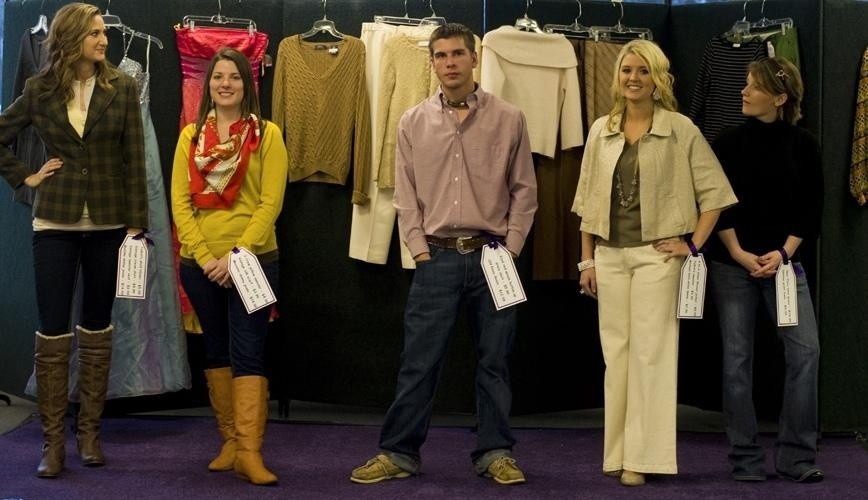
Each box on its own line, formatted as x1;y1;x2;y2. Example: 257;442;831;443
98;0;164;50
543;0;594;38
373;0;438;28
404;0;450;49
749;0;794;31
30;0;48;37
514;0;546;33
298;0;349;41
590;0;653;43
720;0;755;43
183;1;259;38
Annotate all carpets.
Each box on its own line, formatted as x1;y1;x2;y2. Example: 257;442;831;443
0;411;867;499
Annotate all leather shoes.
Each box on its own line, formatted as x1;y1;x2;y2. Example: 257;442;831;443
620;469;647;487
801;472;825;484
731;466;767;482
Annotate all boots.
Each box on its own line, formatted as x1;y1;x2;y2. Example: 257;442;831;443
74;323;118;469
232;374;279;486
32;328;74;480
202;365;235;477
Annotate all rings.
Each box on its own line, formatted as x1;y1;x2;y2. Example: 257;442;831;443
578;288;586;295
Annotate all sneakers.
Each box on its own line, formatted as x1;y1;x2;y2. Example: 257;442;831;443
483;453;527;485
349;451;414;485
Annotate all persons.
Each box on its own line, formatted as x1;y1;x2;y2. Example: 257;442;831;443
704;57;825;484
170;47;290;486
1;3;149;478
569;39;741;488
351;23;540;486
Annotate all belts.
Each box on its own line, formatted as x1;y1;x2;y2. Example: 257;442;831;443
418;233;501;255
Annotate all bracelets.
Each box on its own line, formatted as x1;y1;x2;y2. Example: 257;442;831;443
576;260;595;271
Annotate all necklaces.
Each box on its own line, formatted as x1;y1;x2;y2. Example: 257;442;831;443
614;108;648;209
